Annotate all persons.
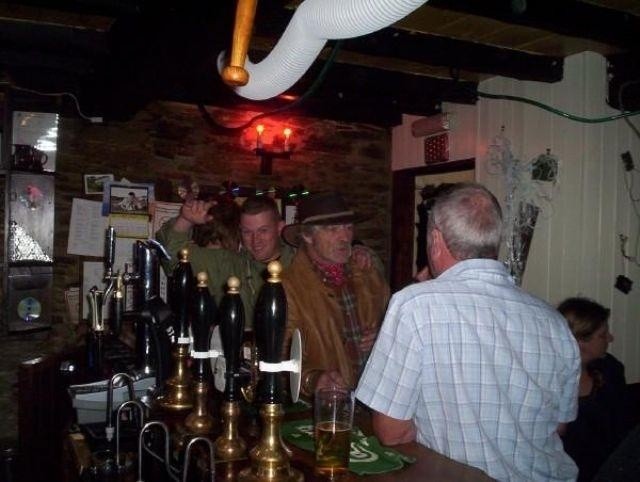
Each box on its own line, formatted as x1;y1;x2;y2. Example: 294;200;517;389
153;193;298;329
120;192;143;211
555;296;627;449
186;186;267;329
279;193;432;418
354;182;582;480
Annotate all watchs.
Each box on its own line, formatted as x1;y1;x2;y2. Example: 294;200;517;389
305;370;321;394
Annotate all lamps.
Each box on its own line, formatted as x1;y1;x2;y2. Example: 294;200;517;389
253;124;294;175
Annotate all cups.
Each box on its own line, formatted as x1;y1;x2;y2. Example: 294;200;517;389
240;340;261;393
89;447;119;482
312;387;353;482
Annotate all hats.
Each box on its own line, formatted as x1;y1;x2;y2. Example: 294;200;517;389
280;187;375;248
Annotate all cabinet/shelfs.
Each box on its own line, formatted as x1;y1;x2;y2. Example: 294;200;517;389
0;168;57;335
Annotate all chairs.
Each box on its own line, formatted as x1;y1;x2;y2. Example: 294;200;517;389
625;382;639;417
